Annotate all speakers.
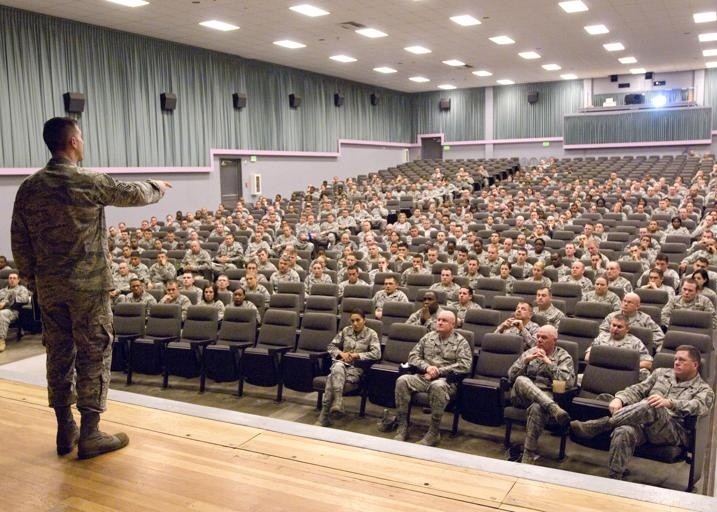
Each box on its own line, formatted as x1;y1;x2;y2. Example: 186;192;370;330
440;98;451;109
528;92;538;102
335;92;345;105
233;93;247;108
609;74;617;81
160;93;177;110
371;93;379;104
64;92;85;113
646;73;653;78
289;94;299;107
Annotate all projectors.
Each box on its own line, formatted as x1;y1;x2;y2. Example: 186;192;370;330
624;93;647;105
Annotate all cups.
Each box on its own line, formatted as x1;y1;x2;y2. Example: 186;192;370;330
553;380;566;393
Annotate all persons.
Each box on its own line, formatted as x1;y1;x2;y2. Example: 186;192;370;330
0;157;717;382
507;324;577;464
314;307;381;427
570;345;716;479
392;311;474;446
11;117;172;459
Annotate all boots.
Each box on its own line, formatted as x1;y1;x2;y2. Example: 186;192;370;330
314;410;334;426
78;414;129;460
570;416;613;439
330;391;346;419
415;425;441;446
0;338;6;352
55;406;80;455
391;421;410;441
548;402;573;434
521;450;535;465
